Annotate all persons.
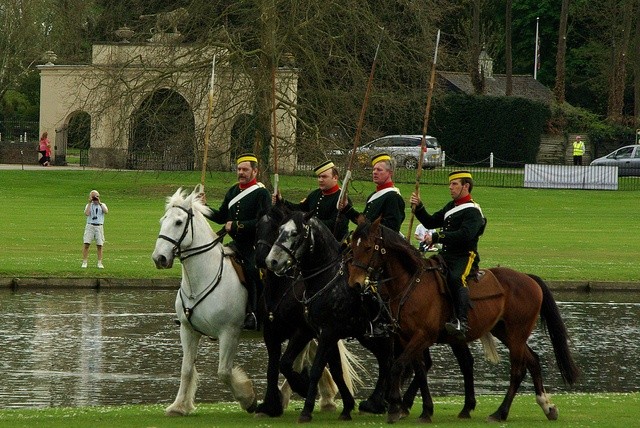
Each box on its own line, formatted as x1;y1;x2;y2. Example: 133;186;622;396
39;132;50;166
174;152;272;330
81;190;108;268
409;171;487;338
572;136;585;166
339;153;405;338
43;143;52;166
414;223;438;252
271;159;353;242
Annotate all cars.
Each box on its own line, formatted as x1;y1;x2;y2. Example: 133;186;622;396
589;145;640;177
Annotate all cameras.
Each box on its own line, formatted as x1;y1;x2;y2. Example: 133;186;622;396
92;196;98;201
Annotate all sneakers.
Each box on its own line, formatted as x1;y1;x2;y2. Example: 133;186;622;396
81;262;87;268
97;263;104;269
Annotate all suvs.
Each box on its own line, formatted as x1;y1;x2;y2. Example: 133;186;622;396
349;134;443;170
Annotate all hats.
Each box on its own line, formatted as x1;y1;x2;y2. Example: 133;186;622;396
237;153;257;164
313;159;334;176
449;170;472;182
371;153;391;166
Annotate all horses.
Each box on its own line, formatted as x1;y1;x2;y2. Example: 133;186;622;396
151;183;372;416
247;201;432;415
347;214;581;426
264;203;476;424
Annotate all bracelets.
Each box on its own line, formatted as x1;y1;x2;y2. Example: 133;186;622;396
99;204;102;206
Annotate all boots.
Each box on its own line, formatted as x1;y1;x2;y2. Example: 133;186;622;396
239;281;263;333
444;287;469;343
